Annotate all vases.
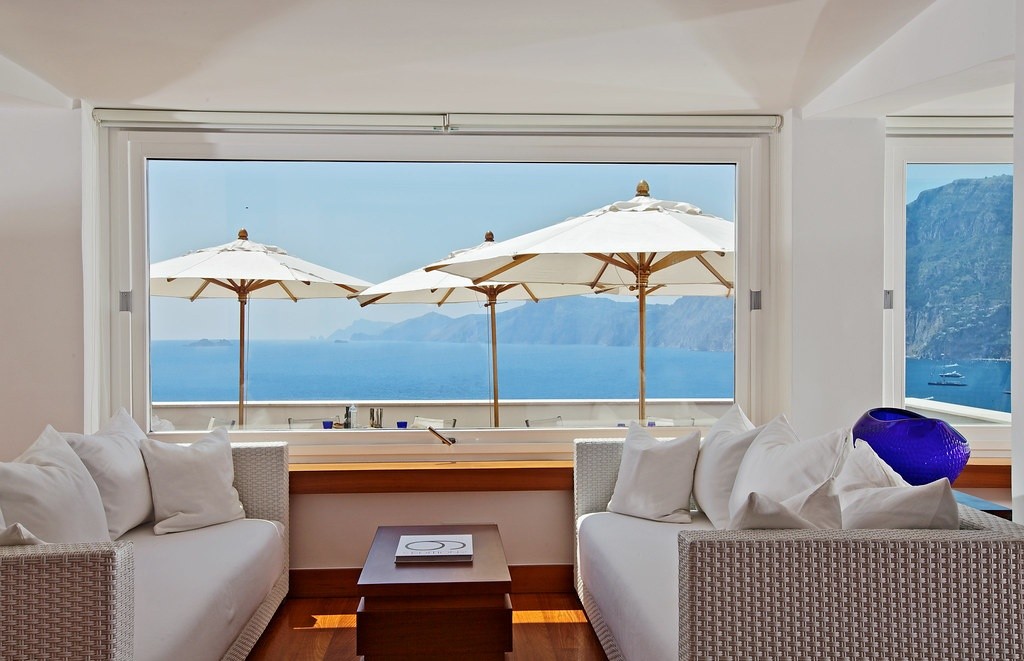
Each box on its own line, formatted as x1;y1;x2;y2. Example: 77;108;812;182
853;407;972;486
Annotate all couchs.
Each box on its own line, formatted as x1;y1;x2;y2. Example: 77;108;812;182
0;440;290;661
572;437;1024;661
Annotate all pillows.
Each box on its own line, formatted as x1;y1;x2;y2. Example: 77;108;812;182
832;438;961;530
605;420;702;524
726;413;854;519
0;424;111;543
59;406;154;540
725;475;843;531
0;507;46;546
139;424;246;536
691;402;767;531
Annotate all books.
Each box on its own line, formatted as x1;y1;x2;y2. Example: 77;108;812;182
394;534;473;563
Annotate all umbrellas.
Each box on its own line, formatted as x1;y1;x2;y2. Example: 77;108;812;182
150;227;378;430
344;229;621;428
422;178;738;426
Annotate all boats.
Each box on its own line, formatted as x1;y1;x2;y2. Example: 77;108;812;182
1001;390;1011;394
951;363;960;367
938;370;962;377
928;341;969;386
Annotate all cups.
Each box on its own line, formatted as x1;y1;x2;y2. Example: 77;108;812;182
397;421;407;428
323;420;333;429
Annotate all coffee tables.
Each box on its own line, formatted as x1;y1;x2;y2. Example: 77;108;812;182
356;524;514;661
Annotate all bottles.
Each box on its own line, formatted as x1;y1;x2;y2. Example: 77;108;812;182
344;407;351;429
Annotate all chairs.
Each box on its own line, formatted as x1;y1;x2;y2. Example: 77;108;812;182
287;415;340;430
413;416;457;427
525;416;564;427
